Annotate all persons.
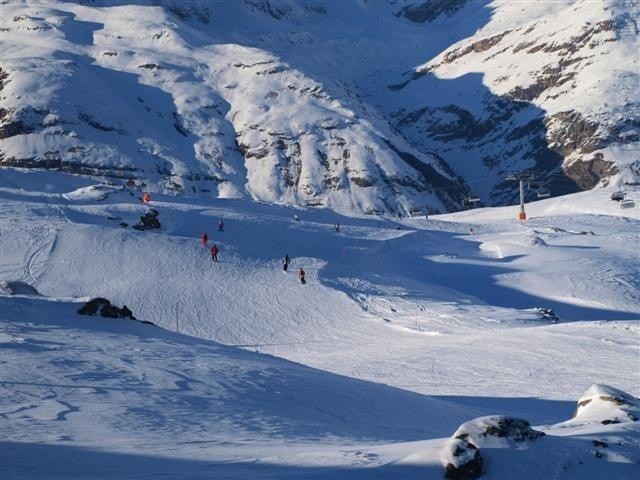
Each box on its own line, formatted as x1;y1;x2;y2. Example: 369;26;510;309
201;231;208;246
282;254;290;270
218;220;224;232
298;267;306;285
294;213;300;223
335;221;339;231
210;244;219;261
